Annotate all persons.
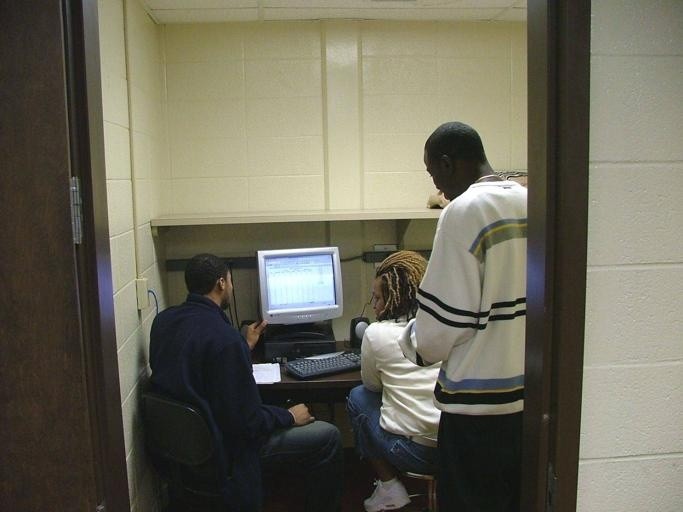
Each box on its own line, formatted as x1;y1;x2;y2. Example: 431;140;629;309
147;252;341;512
344;251;444;512
399;122;528;512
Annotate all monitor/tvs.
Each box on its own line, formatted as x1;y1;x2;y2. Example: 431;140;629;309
257;247;343;338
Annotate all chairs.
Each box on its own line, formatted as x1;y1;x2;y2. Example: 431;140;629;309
139;393;252;512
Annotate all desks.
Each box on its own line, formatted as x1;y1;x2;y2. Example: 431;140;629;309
244;342;364;451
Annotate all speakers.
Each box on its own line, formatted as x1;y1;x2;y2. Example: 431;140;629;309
350;317;370;348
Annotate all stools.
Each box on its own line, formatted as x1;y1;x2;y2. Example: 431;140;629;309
401;470;439;512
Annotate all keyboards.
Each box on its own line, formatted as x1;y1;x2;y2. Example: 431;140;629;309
284;349;362;380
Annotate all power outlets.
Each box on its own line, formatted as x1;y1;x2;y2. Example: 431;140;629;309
137;278;150;310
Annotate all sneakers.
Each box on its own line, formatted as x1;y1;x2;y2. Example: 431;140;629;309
363;475;412;512
